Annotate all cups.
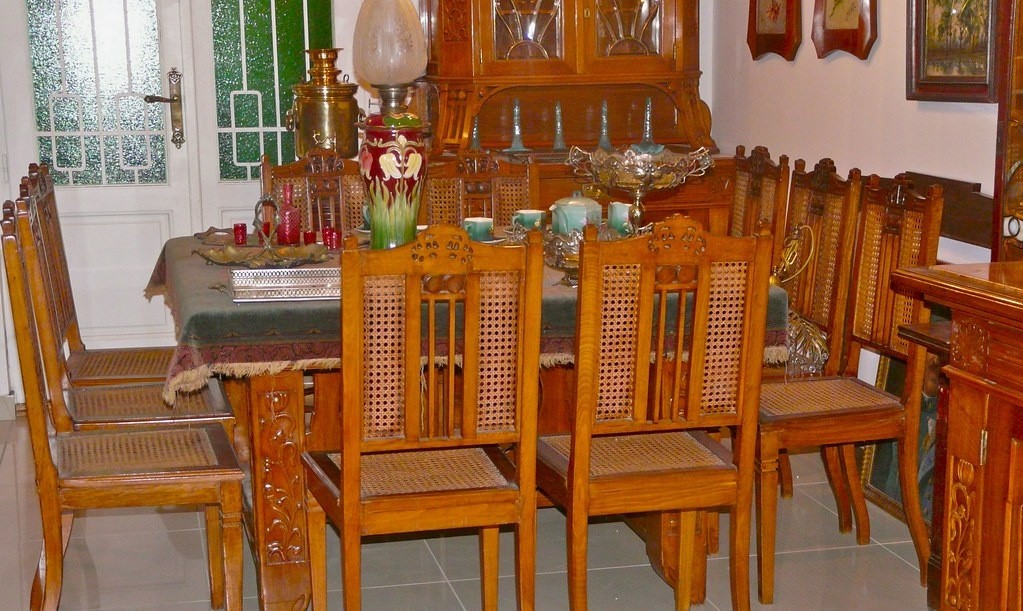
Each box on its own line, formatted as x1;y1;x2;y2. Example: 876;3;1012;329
511;209;546;232
259;222;270;239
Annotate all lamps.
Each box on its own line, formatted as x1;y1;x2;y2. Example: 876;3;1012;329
352;0;423;246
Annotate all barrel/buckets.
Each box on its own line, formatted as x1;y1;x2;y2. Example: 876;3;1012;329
549;191;603;242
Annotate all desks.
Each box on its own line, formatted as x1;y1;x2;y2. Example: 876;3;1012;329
144;225;789;611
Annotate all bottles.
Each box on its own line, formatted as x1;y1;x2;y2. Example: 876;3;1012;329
304;230;316;247
322;227;342;250
463;216;495;242
279;183;300;246
234;223;247;245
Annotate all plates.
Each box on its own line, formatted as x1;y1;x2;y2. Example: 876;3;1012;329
481;236;507;244
503;227;513;234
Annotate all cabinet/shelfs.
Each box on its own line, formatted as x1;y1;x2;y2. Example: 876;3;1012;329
885;262;1023;611
418;0;722;222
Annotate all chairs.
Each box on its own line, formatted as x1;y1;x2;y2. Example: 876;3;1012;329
1;158;248;611
263;150;947;611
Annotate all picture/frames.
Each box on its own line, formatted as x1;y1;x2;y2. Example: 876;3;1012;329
810;0;878;59
859;259;952;540
747;0;802;65
903;0;998;102
